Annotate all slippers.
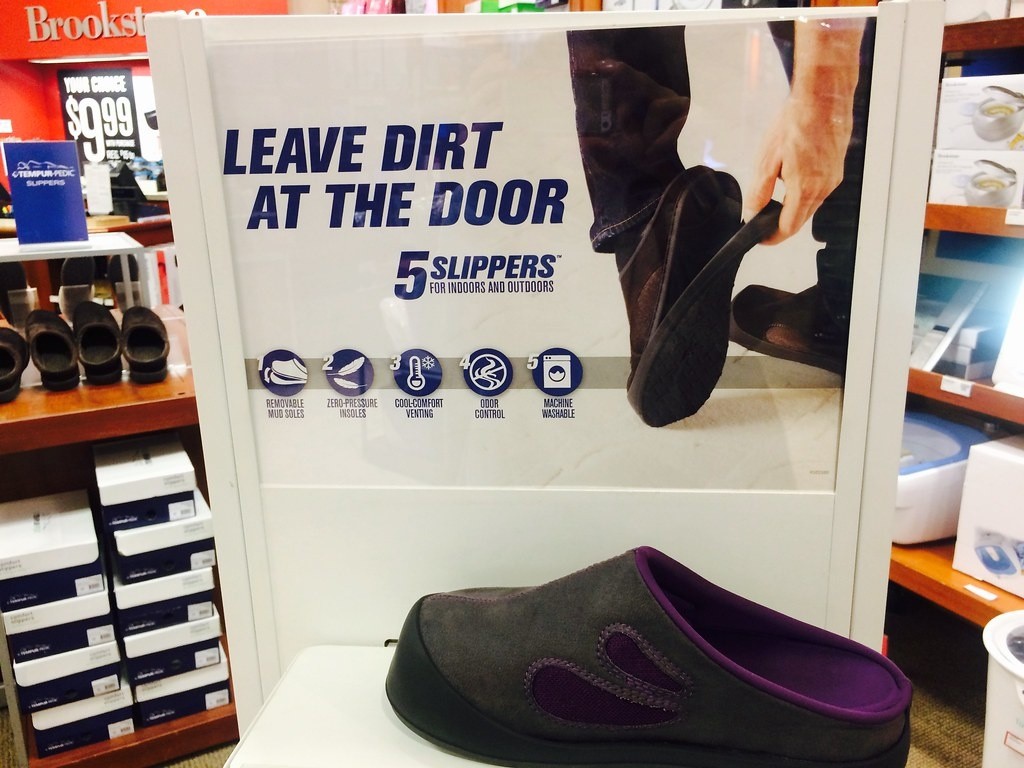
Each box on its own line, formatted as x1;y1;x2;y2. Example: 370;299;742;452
727;283;851;376
384;546;913;768
618;163;785;427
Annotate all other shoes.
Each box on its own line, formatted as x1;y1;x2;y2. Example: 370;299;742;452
73;301;123;384
121;306;170;384
0;326;30;405
0;261;29;329
24;309;81;391
107;252;140;312
60;255;94;323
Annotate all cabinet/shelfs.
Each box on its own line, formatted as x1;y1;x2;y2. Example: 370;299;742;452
0;192;198;454
888;17;1024;625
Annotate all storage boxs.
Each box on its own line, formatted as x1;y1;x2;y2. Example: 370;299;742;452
112;484;216;585
3;572;115;663
13;640;121;715
134;641;230;727
935;75;1024;151
122;603;222;687
928;151;1024;209
952;433;1024;599
92;433;196;533
31;676;136;758
113;567;213;635
0;482;105;612
